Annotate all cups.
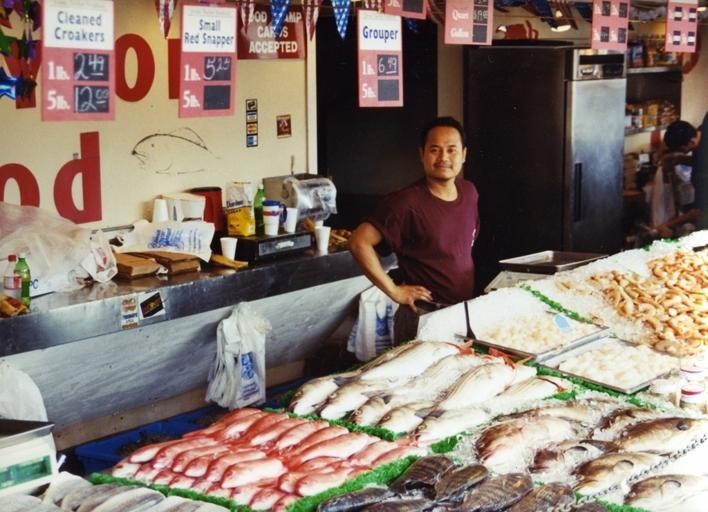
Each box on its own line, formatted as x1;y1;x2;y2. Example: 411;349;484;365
220;237;238;261
314;226;331;251
153;200;169;222
262;201;298;236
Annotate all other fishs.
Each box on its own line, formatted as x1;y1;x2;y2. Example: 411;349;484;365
0;334;706;512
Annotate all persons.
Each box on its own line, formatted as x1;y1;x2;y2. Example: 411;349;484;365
347;114;483;347
656;110;708;233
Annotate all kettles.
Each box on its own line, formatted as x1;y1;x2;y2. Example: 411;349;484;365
162;192;206;222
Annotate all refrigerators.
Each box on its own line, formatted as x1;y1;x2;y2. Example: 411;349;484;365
462;38;629;253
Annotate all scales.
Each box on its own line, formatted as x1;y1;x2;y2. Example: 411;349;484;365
0;418;57;496
484;250;609;294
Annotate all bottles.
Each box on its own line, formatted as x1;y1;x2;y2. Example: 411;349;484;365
253;183;267;236
3;252;31;310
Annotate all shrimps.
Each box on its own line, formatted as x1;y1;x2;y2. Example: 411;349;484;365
587;248;707;357
